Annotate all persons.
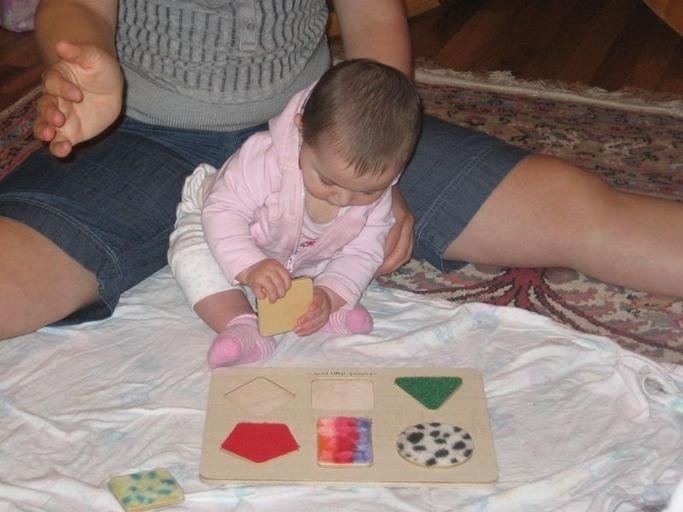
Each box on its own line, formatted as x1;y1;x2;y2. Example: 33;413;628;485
0;0;681;348
164;56;426;372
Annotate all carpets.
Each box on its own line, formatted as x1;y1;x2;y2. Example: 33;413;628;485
1;67;682;363
